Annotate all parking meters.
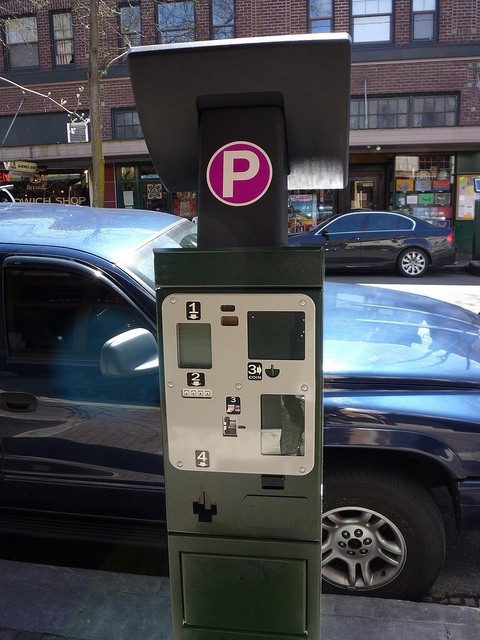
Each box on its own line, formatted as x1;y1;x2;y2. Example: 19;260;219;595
128;31;352;639
1;204;480;600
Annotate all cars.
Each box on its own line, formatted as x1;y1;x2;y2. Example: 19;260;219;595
288;210;458;278
